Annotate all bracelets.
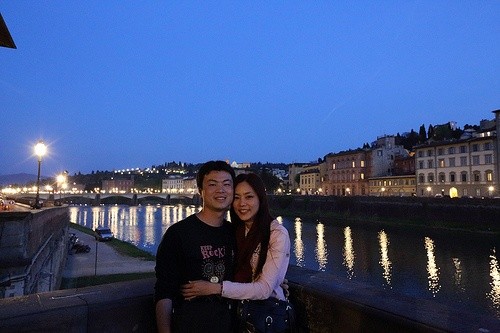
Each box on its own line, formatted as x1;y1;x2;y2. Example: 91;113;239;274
220;282;223;295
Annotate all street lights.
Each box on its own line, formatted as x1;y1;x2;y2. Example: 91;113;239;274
35;143;46;200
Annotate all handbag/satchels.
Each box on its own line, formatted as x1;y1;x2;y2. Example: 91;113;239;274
242;297;291;333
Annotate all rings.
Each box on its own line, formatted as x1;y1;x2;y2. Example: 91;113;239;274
190;284;191;288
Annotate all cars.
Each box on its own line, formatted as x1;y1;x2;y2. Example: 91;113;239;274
95;228;113;240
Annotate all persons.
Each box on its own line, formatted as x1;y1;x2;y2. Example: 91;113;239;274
153;160;290;333
70;233;91;253
29;198;41;210
179;173;294;333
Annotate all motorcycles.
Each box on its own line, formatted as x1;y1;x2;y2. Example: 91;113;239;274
74;238;91;252
